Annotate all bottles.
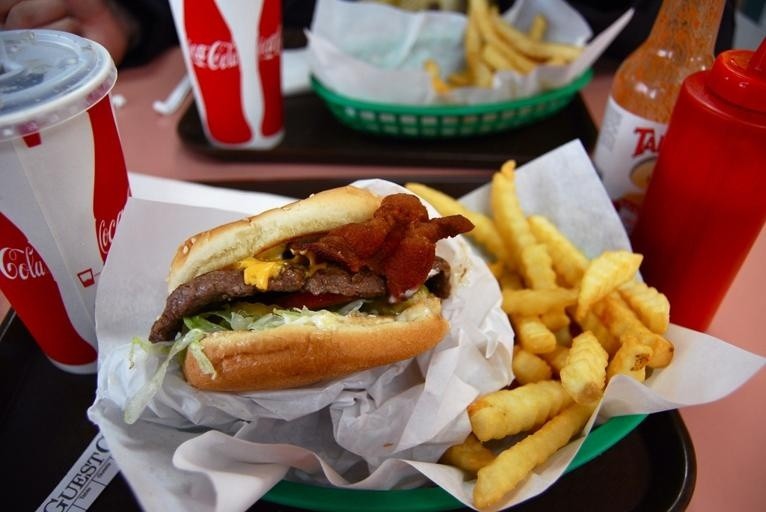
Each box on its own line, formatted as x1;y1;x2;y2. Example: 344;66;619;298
593;0;729;205
636;36;766;332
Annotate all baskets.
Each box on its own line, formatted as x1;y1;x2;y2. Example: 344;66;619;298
308;60;595;140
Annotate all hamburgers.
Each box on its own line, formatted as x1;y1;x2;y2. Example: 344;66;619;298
124;186;476;428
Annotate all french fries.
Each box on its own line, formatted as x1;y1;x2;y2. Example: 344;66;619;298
424;0;583;97
406;159;674;510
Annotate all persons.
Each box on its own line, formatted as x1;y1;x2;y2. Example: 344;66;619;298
1;0;742;109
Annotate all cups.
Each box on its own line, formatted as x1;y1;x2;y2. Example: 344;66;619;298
0;28;141;378
170;2;285;151
